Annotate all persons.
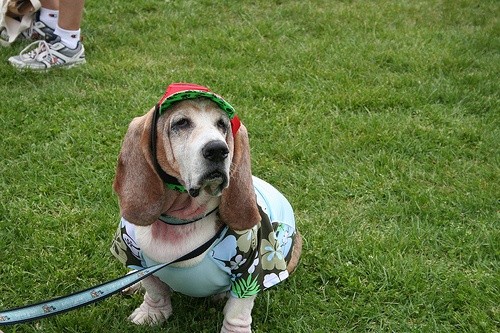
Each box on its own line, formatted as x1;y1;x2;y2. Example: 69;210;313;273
8;0;88;73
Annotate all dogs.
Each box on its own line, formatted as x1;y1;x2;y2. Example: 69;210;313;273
106;83;301;333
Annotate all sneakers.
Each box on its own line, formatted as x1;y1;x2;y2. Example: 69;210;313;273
1;21;55;40
8;34;87;73
